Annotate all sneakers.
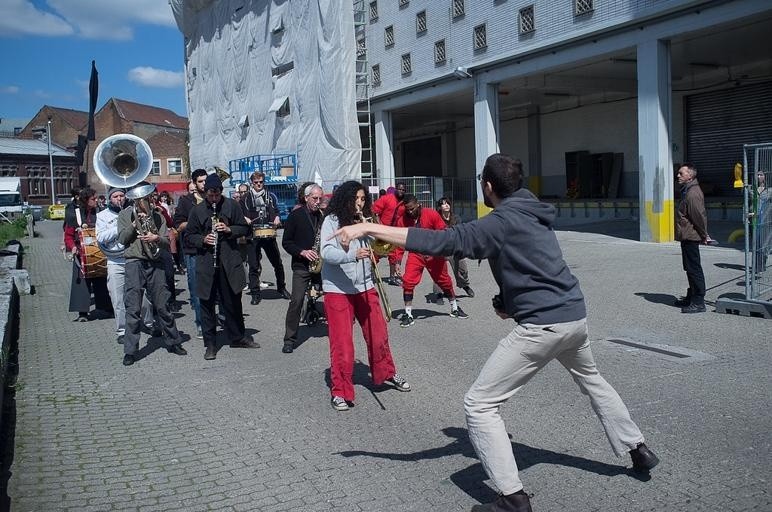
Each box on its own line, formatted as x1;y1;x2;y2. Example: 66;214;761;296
260;282;268;288
682;302;706;313
78;313;89;322
384;374;411;392
196;325;204;339
333;395;349;411
675;297;691;306
400;313;415;327
449;306;468;318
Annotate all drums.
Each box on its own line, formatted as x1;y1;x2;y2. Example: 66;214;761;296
74;228;108;277
252;223;277;239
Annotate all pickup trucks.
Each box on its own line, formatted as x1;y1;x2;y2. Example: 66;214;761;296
20;205;43;227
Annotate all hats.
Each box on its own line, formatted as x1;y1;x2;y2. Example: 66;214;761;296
204;173;223;192
109;187;126;198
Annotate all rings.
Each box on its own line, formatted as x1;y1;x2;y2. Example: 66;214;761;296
342;239;346;242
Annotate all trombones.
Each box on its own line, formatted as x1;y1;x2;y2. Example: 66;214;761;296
354;207;392;323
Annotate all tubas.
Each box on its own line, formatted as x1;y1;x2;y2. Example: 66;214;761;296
93;134;153;210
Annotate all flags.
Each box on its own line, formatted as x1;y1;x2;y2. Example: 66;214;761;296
86;59;99;142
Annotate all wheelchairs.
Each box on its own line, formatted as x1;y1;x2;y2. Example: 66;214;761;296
298;274;355;327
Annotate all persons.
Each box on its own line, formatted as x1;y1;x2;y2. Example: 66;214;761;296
327;153;660;511
386;194;469;328
365;183;409;286
280;184;323;352
674;163;712;315
293;182;315;212
62;168;290;365
744;169;771;273
431;197;475;298
323;181;412;410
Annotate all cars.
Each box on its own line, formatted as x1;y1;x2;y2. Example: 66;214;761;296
292;194;333;212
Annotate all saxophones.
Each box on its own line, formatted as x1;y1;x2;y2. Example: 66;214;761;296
308;205;325;272
124;184;163;259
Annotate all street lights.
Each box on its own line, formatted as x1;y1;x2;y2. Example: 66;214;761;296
47;114;55;205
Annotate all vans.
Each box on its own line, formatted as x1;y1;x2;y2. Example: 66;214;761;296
0;191;24;222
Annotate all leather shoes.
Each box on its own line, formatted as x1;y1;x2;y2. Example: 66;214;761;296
463;286;474;297
251;292;262;305
118;325;187;365
205;343;217;359
230;334;261;348
277;288;291;299
389;279;401;286
472;492;532;512
629;443;658;480
283;344;293;353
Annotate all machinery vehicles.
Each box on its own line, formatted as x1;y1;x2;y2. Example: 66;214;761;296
229;154;297;228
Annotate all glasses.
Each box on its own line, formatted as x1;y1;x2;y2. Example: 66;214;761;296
254;181;263;184
478;174;483;180
